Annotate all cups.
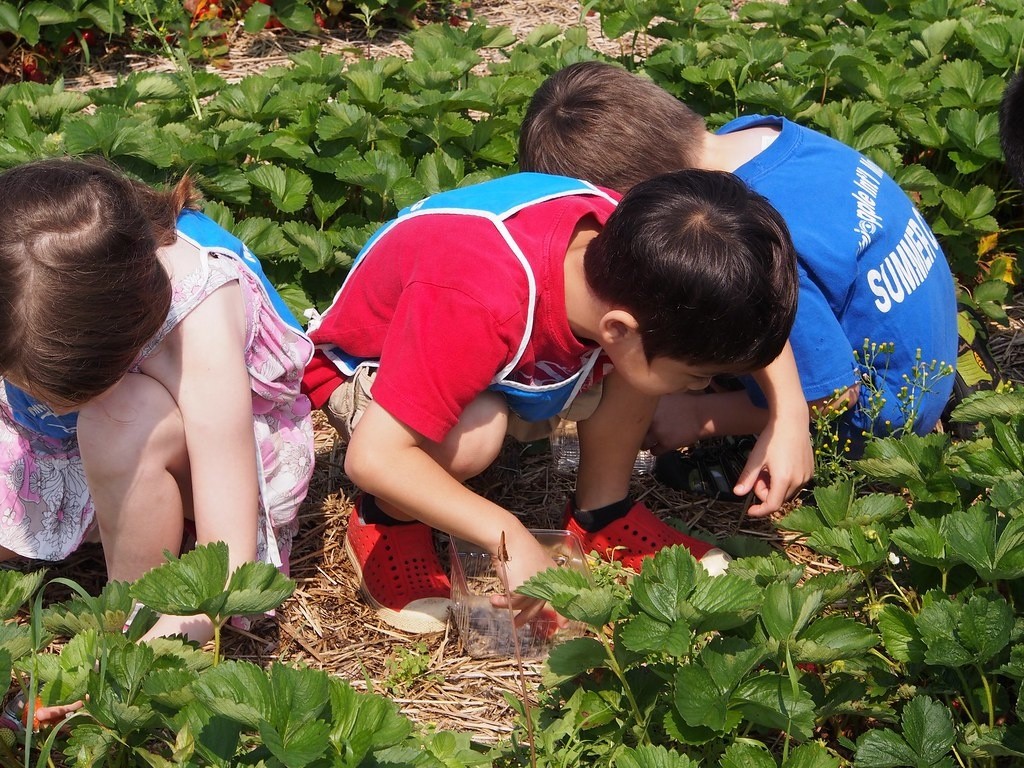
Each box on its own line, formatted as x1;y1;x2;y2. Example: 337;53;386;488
551;426;579;473
633;450;656;476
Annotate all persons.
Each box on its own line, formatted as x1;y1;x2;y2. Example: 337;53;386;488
0;155;316;749
300;169;814;633
519;60;1020;501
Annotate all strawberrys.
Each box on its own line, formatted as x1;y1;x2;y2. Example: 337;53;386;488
21;0;334;82
21;697;46;730
528;610;558;641
794;662;820;675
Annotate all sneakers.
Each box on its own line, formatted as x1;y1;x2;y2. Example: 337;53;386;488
653;435;764;504
560;494;733;578
343;494;451;634
